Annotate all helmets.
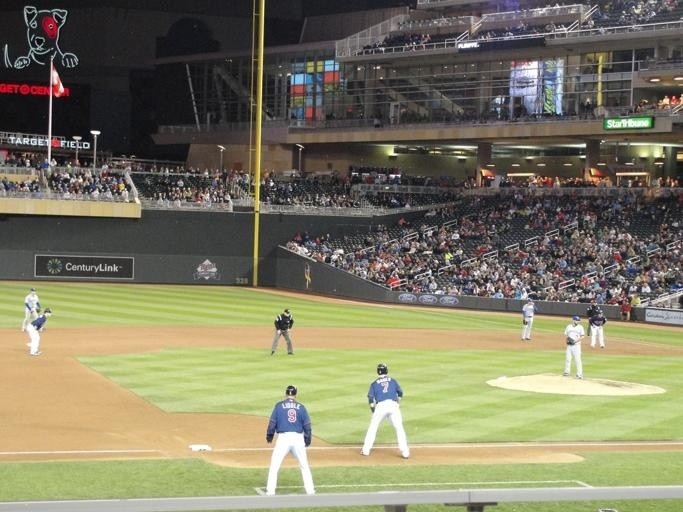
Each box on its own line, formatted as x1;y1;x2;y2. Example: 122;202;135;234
377;363;388;375
286;386;297;396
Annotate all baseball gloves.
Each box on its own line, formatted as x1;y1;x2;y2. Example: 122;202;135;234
566;337;575;345
523;320;528;324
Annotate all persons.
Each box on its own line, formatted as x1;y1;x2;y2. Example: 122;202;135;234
360;363;409;459
586;300;600;336
0;149;511;214
589;307;605;348
290;93;682;128
285;168;682;320
22;288;43;332
355;2;682;57
26;308;51;356
266;385;315;496
563;316;585;379
522;299;537;340
271;309;294;356
